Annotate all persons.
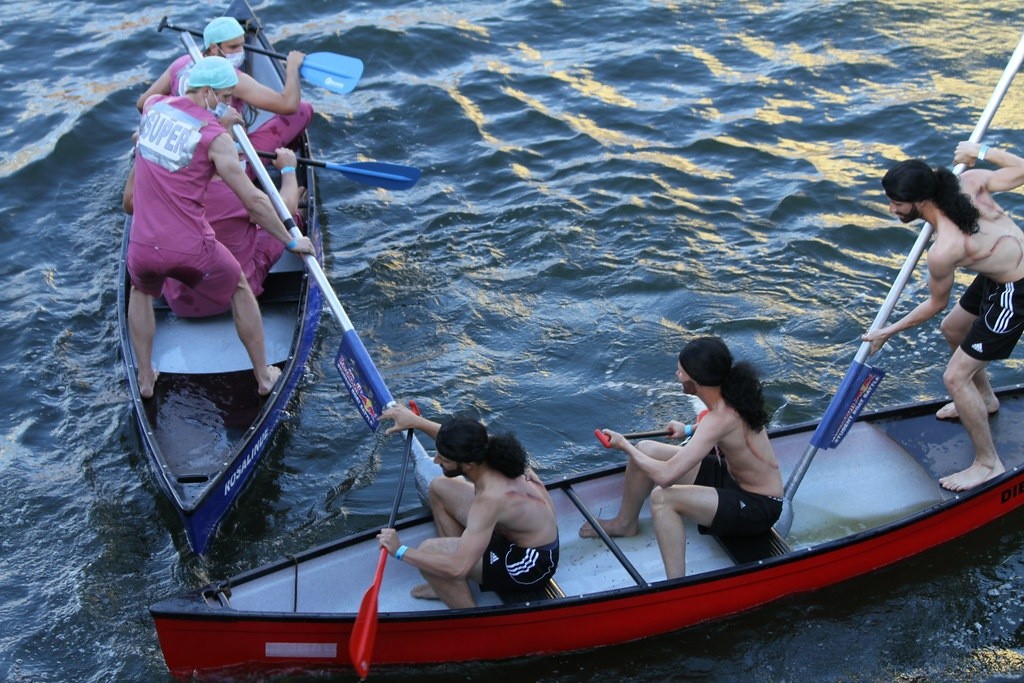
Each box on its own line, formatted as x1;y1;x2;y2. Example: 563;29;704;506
122;16;317;397
377;403;560;610
579;336;783;581
859;142;1024;492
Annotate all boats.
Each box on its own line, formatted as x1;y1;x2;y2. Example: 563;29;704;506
147;380;1024;674
116;2;325;557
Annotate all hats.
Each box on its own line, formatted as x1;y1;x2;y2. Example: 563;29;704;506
203;15;244;50
185;56;239;90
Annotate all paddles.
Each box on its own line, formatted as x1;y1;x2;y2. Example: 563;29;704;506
236;147;421;193
593;428;688;450
158;14;366;96
347;400;411;683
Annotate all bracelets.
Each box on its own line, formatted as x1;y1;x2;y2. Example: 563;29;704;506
978;145;988;160
287;239;296;250
685;425;691;437
280;166;295;174
396;545;408;561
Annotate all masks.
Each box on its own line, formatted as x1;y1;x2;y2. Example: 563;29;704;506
212;42;245;68
203;87;228;119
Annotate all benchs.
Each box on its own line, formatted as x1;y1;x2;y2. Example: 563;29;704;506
715;529;795;567
493;578;567;605
268;247;306;275
